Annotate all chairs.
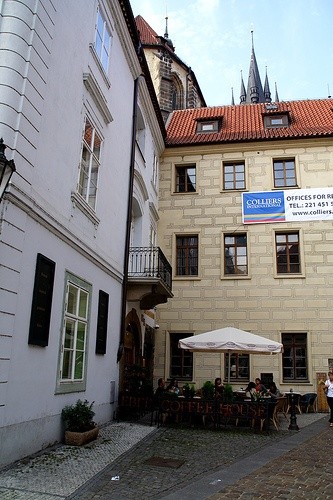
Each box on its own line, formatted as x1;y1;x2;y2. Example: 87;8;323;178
148;391;319;434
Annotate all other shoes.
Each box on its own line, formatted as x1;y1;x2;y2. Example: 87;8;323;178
330;423;333;426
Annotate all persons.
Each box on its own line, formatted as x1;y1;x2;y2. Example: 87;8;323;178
244;378;280;435
213;377;225;393
154;378;180;427
322;371;333;427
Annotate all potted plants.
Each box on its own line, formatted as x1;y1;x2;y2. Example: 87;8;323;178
61;398;101;447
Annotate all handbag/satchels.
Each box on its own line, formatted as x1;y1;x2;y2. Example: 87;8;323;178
323;387;328;394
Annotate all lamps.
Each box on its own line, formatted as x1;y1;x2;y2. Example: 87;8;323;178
154;324;160;330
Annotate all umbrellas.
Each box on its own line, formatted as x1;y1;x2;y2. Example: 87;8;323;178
178;327;284;385
